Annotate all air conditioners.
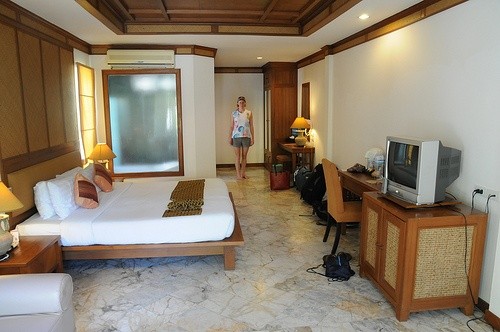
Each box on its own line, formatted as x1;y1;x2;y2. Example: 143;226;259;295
106;50;175;69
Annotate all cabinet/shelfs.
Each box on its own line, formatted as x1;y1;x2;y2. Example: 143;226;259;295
263;61;298;172
359;192;488;322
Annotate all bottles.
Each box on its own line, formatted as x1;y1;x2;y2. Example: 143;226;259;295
10;230;20;246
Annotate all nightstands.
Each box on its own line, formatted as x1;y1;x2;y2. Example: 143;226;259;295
0;235;64;275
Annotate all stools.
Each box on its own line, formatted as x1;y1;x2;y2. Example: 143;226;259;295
276;155;302;174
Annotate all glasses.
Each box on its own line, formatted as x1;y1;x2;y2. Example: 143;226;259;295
238;96;245;99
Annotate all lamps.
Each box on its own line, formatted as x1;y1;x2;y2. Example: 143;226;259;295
290;117;311;146
0;181;24;262
87;143;117;168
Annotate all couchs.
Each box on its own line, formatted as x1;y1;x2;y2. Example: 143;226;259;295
0;273;75;332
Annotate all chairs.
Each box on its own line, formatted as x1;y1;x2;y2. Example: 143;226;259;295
322;159;361;254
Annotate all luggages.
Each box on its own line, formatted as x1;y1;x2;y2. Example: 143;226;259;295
300;163;326;207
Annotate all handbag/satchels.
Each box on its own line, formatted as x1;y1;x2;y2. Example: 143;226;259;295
270;162;290;190
321;251;355;282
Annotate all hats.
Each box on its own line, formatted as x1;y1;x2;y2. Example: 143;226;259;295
236;96;246;107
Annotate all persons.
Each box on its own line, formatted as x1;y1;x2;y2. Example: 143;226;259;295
229;97;255;181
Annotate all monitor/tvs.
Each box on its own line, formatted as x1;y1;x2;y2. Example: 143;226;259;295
381;136;462;205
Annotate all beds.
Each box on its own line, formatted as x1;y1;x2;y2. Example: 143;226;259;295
0;140;245;270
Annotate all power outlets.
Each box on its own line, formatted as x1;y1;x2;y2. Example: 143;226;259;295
474;185;500;202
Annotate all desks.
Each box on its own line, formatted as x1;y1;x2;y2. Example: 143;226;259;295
337;171;380;235
280;143;315;186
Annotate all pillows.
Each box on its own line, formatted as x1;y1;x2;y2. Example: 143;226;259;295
33;161;112;219
